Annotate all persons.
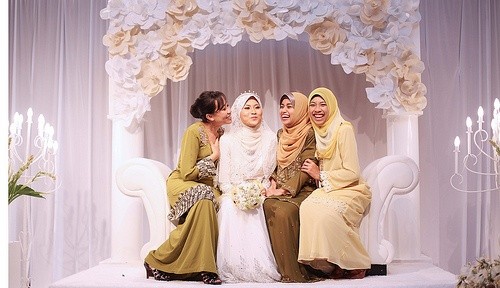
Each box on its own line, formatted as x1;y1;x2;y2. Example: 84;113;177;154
297;87;371;279
262;91;325;283
144;91;232;284
215;90;282;286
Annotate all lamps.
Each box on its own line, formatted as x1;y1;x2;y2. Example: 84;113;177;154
450;98;500;193
8;107;59;288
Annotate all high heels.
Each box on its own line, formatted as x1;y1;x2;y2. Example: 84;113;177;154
144;261;173;281
200;271;222;284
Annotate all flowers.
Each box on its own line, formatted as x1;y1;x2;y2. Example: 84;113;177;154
231;179;266;212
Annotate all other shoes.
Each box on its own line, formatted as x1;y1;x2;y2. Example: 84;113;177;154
330;265;366;279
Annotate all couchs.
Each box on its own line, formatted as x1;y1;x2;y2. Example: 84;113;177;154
115;155;420;278
